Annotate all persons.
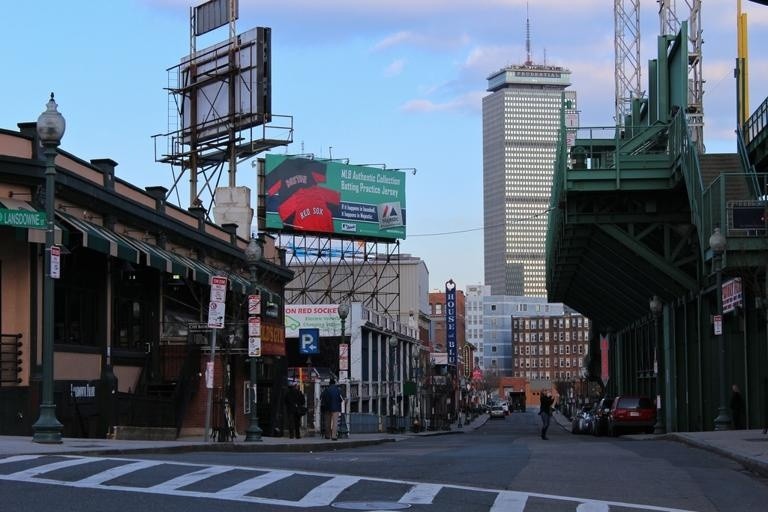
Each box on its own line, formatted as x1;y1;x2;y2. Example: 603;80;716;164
320;379;345;440
538;387;556;440
286;384;306;439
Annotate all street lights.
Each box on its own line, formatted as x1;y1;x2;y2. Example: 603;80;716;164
338;300;349;344
31;93;66;443
649;295;665;434
411;346;420;425
709;228;732;431
429;357;436;428
389;332;398;426
243;233;263;442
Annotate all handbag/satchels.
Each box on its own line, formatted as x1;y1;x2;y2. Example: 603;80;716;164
294;406;308;416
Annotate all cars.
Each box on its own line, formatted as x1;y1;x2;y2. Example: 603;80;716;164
488;392;526;420
570;395;657;438
